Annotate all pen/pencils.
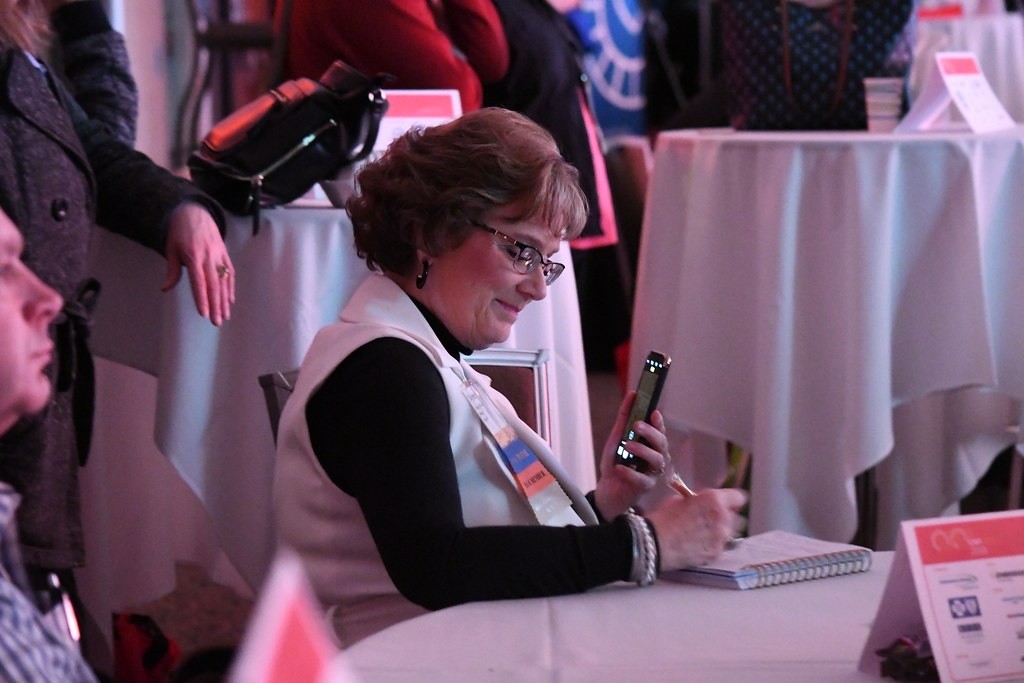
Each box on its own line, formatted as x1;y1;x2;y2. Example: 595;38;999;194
665;474;738;550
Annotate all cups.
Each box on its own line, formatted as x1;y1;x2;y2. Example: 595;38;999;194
865;78;904;133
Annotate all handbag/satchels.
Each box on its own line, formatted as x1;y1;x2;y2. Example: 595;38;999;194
185;60;397;236
720;0;918;132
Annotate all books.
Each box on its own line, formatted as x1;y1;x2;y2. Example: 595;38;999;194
672;528;874;590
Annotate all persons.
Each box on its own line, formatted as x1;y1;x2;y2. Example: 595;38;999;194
0;0;235;683
0;211;102;682
274;106;753;652
277;0;624;250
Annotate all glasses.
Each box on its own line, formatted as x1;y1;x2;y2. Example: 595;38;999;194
472;221;565;286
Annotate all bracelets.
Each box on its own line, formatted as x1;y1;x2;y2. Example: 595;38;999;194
615;511;662;587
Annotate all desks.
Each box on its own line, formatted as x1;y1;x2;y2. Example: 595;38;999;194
330;551;899;683
76;168;596;673
629;127;1024;545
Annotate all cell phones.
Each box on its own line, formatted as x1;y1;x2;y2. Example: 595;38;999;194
614;351;672;472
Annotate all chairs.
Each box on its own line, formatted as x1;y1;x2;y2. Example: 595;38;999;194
256;346;553;458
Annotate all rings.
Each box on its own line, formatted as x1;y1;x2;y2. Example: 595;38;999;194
648;459;666;474
216;264;232;281
